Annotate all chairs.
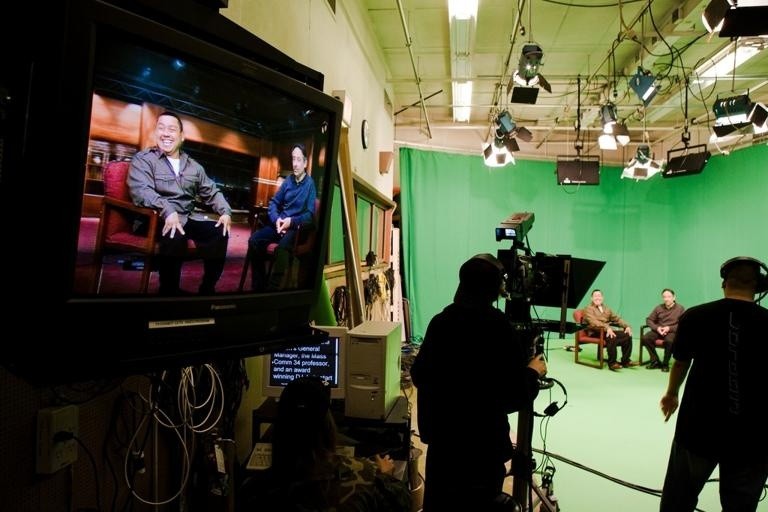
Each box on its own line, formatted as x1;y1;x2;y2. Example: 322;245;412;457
572;309;628;369
637;325;672;368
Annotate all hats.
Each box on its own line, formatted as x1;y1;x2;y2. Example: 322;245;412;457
279;376;331;420
454;254;502;304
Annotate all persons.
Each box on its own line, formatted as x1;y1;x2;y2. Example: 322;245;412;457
582;289;641;369
410;258;540;511
644;289;685;372
235;378;337;511
249;142;316;290
659;257;767;512
125;112;233;296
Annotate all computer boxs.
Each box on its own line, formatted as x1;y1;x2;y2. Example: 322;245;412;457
345;320;404;422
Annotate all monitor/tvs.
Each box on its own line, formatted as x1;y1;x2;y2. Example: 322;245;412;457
0;2;345;378
262;322;349;402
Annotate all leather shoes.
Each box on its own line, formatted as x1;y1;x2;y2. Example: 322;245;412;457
663;362;669;372
623;360;639;368
609;363;621;370
646;361;661;369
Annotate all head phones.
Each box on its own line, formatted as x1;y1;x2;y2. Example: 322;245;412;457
531;377;567;416
720;257;767;305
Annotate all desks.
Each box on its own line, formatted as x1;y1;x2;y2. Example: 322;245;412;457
251;396;425;512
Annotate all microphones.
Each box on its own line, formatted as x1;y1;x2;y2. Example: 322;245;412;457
532;410;548;418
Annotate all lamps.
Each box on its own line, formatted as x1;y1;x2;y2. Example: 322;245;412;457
448;0;476;125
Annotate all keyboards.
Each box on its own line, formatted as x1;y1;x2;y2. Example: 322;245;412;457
246;439;355;474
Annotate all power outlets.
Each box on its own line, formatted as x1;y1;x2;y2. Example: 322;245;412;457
35;404;79;474
